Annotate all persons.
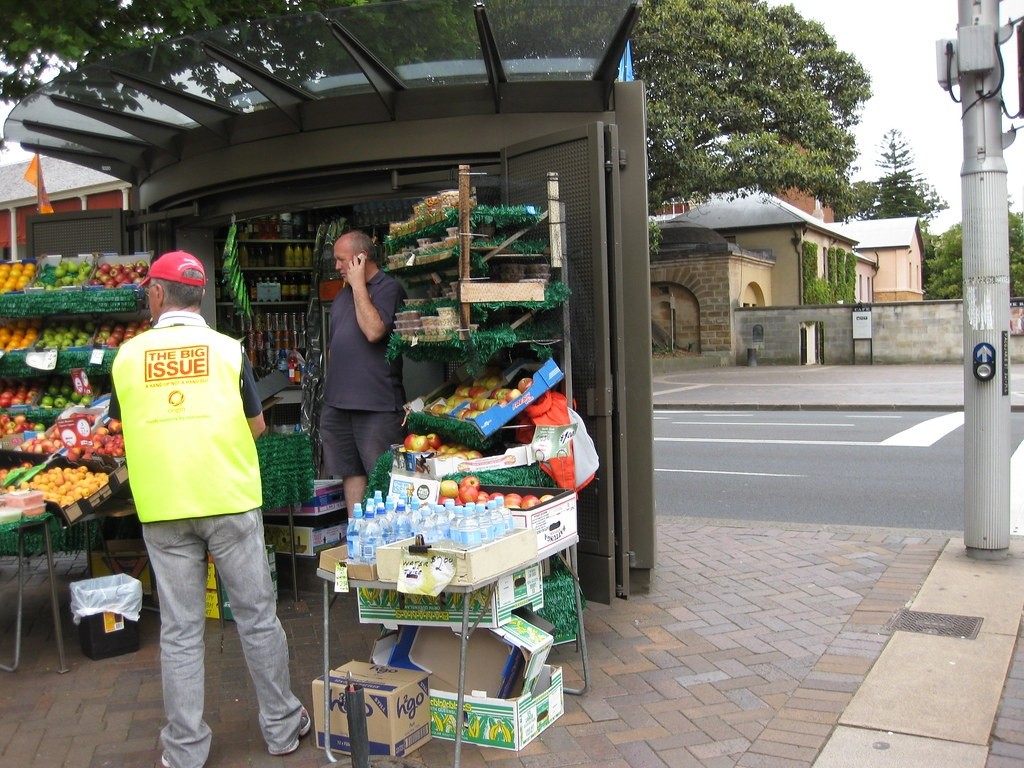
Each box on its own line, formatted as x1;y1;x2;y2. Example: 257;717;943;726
108;250;310;768
319;230;405;518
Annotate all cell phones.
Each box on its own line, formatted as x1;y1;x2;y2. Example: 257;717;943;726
352;253;367;266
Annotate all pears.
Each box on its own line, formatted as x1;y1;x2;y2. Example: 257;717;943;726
36;321;94;351
46;256;91;290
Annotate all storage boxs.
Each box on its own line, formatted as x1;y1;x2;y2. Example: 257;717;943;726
255;369;292;403
262;523;348;556
423;357;566;439
90;542;154;596
357;561;543;629
0;249;158;527
457;483;578;551
408;613;554;700
429;665;565;752
221;571;279;622
392;444;527;481
102;516;146;551
311;659;433;758
262;480;347;517
375;524;540;587
318;544;380;581
219;542;277;590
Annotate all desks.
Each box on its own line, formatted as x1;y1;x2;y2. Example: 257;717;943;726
316;533;590;768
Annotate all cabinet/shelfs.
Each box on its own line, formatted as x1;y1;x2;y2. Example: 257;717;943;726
384;165;573;411
212;236;316;308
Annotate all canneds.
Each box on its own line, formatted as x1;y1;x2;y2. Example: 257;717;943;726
237;312;306;368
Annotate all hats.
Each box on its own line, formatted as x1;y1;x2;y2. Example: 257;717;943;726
136;249;206;288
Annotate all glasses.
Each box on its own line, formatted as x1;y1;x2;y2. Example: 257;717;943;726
144;283;155;296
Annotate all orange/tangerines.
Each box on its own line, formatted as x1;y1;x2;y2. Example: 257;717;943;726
0;320;41;352
0;263;35;292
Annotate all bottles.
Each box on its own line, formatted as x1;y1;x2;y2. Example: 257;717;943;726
360;511;381;564
236;212;318;239
451;502;495;548
486;496;514;538
280;271;310;301
416;507;437;544
399;491;410;511
278;350;289;379
376;508;394;545
394;505;410;542
287;359;296;381
429;499;456;540
214;239;313;267
246;274;281;301
385;496;395;521
408;499;421;537
373;491;383;504
365;499;375;508
347;502;365;565
215;277;233;302
296;364;301;385
352;199;422;227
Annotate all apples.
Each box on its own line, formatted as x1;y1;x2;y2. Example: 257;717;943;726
0;378;124;506
432;377;533;421
95;318;151;347
91;260;148;288
438;476;554;508
398;434;484;459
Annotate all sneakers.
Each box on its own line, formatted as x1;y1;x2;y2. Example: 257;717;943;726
162;754;172;768
267;704;311;756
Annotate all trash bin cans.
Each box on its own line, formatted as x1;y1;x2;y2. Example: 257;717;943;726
70;570;143;661
